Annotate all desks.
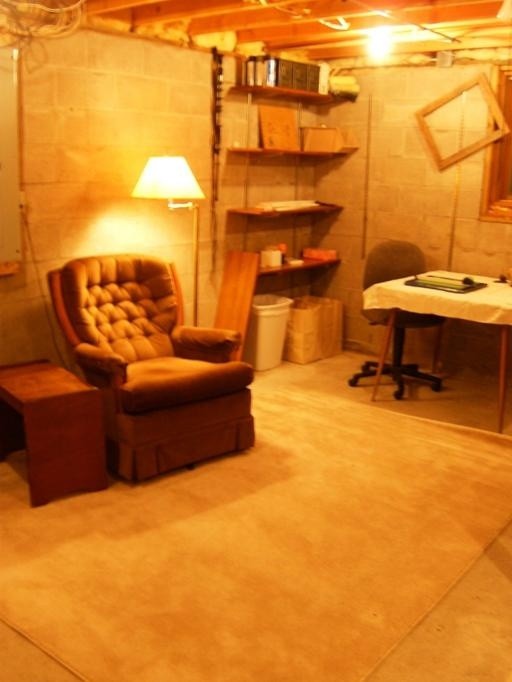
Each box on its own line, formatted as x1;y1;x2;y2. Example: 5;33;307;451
364;269;512;434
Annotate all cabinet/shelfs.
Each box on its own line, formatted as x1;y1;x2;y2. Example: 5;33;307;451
225;79;352;275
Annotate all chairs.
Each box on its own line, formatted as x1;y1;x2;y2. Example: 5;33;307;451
347;240;443;400
48;251;258;483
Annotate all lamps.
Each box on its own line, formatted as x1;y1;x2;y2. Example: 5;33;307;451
131;156;208;327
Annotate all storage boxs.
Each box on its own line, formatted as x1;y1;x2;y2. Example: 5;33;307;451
299;126;338;152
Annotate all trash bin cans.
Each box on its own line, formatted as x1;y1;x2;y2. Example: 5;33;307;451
245;294;294;371
